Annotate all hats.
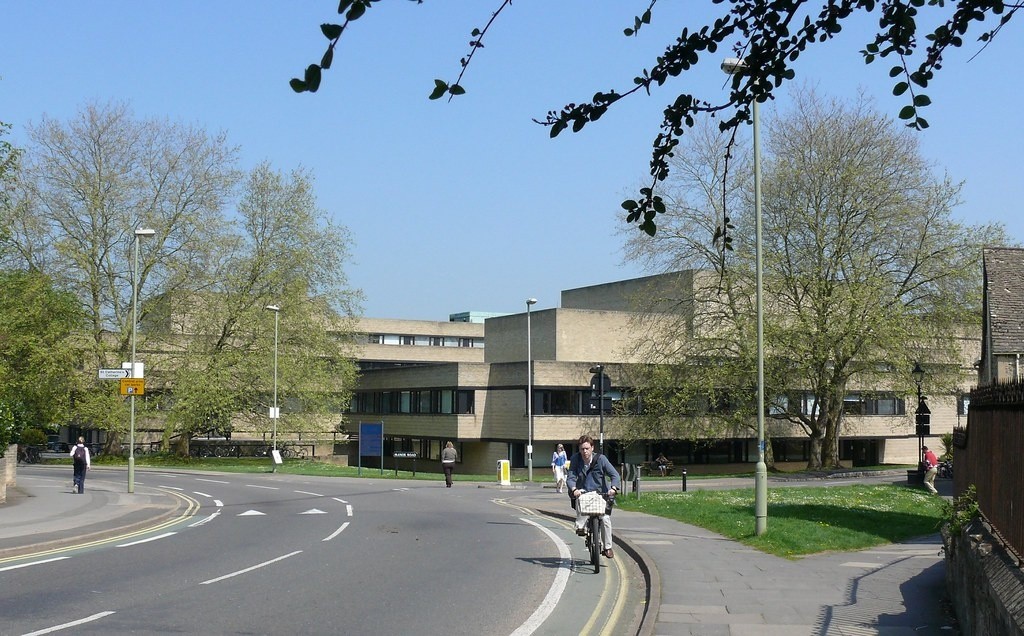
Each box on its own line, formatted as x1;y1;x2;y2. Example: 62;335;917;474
659;453;662;456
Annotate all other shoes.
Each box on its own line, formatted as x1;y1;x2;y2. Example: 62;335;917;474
576;529;584;534
556;488;562;493
447;484;451;487
74;485;79;494
604;548;614;559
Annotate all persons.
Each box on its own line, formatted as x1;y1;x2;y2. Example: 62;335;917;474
550;443;567;493
922;446;938;495
441;441;457;487
566;435;621;558
70;436;91;494
656;452;670;477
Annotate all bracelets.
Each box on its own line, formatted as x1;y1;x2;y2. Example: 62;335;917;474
611;487;617;493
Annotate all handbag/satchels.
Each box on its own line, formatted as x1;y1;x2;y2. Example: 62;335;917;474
563;466;568;474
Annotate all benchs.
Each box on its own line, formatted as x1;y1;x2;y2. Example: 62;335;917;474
640;460;675;476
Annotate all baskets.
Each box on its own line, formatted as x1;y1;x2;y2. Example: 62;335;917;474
576;498;606;515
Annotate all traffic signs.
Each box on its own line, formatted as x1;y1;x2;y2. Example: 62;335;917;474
120;378;145;395
98;368;132;379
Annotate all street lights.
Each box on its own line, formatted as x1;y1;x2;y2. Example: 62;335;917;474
911;363;927;467
526;296;537;482
589;364;606;456
127;227;155;494
719;57;768;538
265;304;279;476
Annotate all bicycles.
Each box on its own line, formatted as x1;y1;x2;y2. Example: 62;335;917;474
937;457;953;479
572;491;617;574
16;445;43;464
88;442;161;457
254;441;309;460
188;442;244;458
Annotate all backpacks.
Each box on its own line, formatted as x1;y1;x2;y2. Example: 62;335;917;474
74;446;86;463
